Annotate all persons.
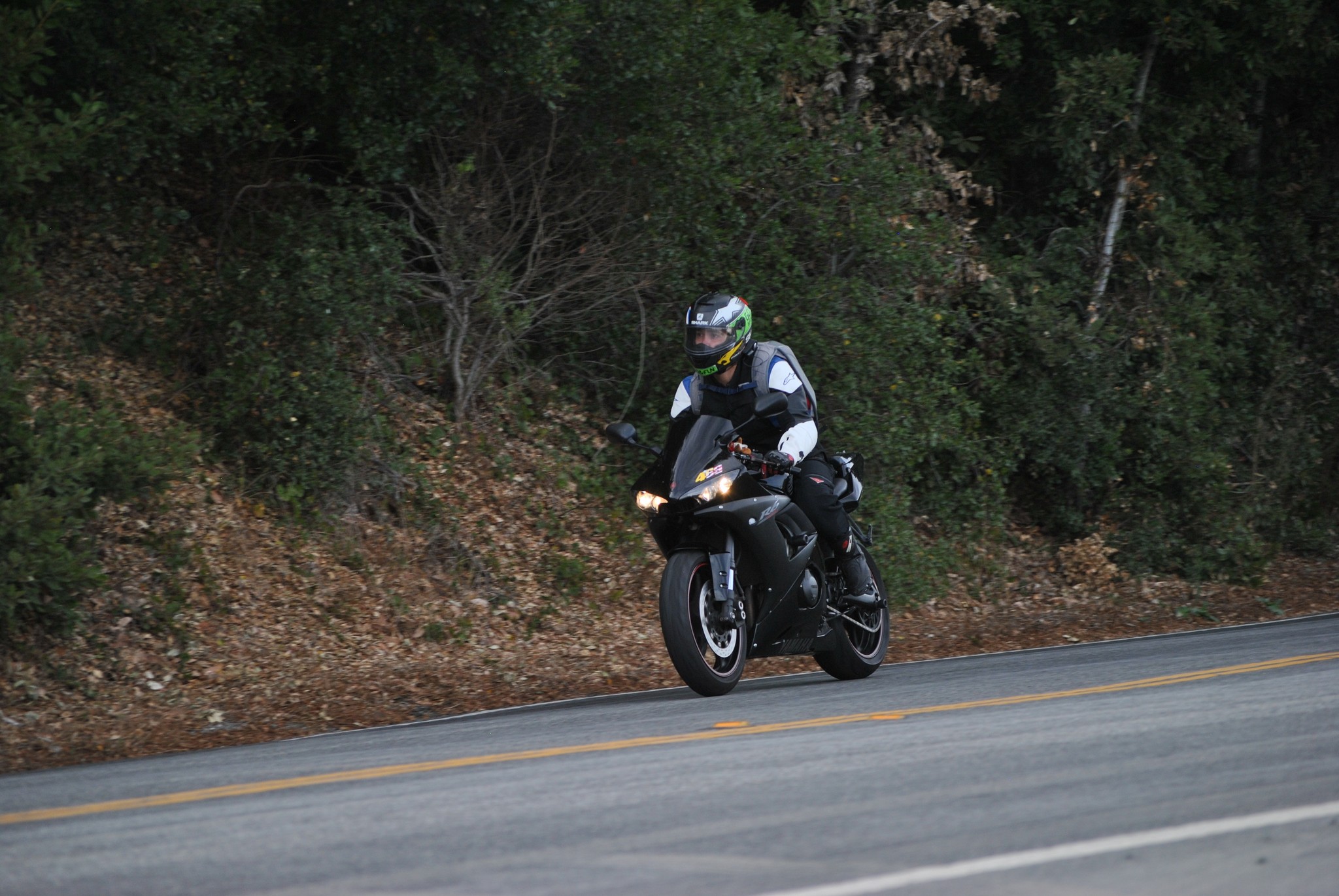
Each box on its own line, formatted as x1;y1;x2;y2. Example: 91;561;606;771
631;289;882;606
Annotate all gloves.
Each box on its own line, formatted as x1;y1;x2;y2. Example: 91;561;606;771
761;450;794;479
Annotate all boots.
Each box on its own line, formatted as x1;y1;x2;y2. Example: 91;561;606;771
829;520;872;597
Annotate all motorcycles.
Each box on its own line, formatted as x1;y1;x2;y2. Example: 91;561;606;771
605;393;890;697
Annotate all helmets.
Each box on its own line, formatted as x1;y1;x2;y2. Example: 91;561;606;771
684;292;752;375
